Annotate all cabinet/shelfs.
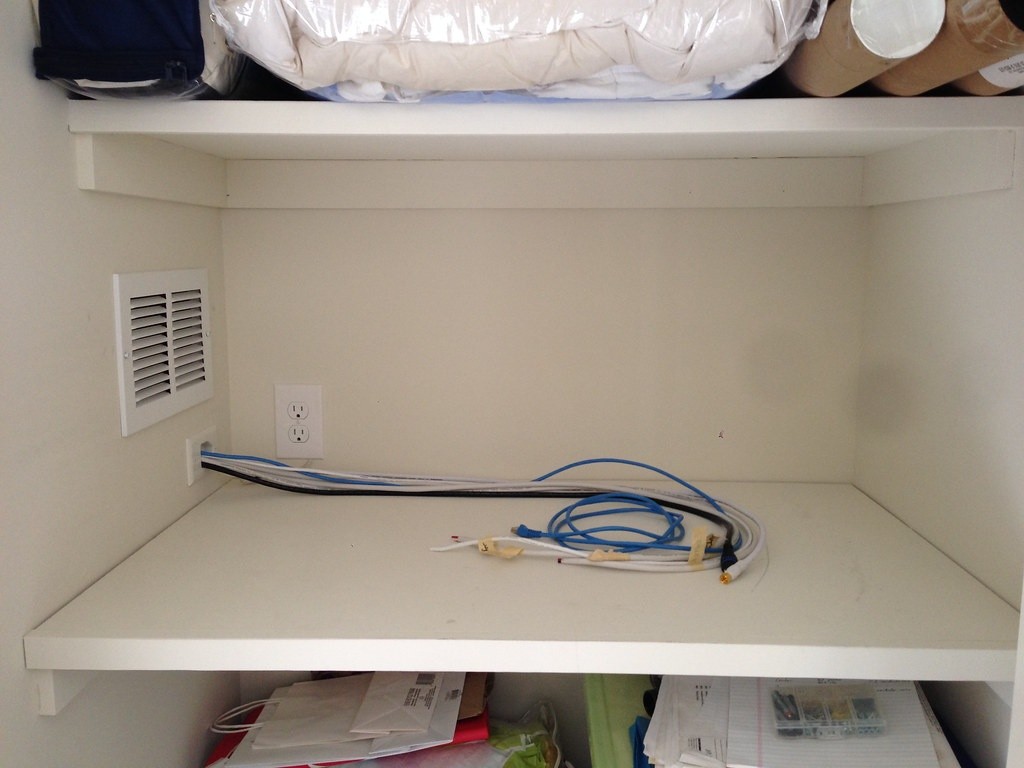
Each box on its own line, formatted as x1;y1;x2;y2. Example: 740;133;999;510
0;0;1024;768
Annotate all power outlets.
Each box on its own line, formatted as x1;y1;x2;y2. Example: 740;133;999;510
273;383;326;461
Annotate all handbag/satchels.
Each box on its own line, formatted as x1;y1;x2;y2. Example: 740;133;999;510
210;672;466;768
